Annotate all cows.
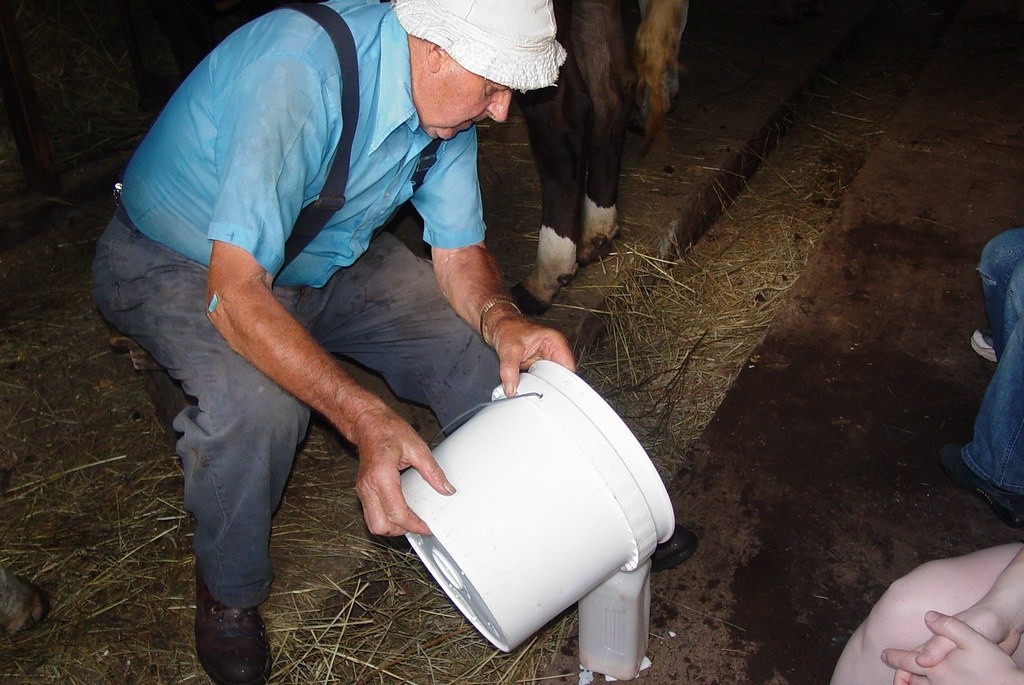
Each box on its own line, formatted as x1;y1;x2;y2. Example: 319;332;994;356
509;1;692;318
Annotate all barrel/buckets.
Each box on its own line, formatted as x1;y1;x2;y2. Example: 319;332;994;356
401;360;675;652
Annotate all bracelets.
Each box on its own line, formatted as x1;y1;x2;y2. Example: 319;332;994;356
479;298;524;345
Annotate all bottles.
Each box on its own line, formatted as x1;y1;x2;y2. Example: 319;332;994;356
578;557;651;680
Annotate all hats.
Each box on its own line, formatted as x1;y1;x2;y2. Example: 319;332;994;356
394;0;567;92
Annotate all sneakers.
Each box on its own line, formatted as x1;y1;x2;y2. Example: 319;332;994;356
969;328;998;363
194;558;272;685
651;524;699;573
937;442;1024;529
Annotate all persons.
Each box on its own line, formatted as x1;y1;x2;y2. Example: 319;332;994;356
830;224;1024;685
86;0;696;685
